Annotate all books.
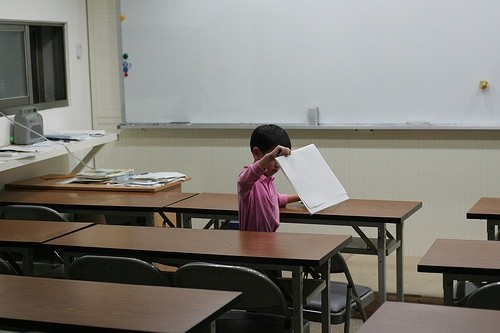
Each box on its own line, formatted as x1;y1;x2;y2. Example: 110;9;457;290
76;168;133;183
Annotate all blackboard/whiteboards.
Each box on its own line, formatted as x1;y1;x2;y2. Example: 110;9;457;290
116;0;500;130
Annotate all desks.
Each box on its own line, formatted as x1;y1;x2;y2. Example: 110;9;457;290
0;219;96;277
416;237;500;305
0;188;200;227
465;197;500;241
5;173;180;271
0;132;117;173
164;193;424;308
353;300;500;333
44;223;352;333
0;273;243;333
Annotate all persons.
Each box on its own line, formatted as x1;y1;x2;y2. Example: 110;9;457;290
237;125;301;278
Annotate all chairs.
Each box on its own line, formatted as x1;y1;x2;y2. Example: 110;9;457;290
0;203;374;333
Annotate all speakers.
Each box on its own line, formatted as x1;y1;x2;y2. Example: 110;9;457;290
13;108;43;144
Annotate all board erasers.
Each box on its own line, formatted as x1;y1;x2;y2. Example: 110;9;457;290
308;107;318;125
